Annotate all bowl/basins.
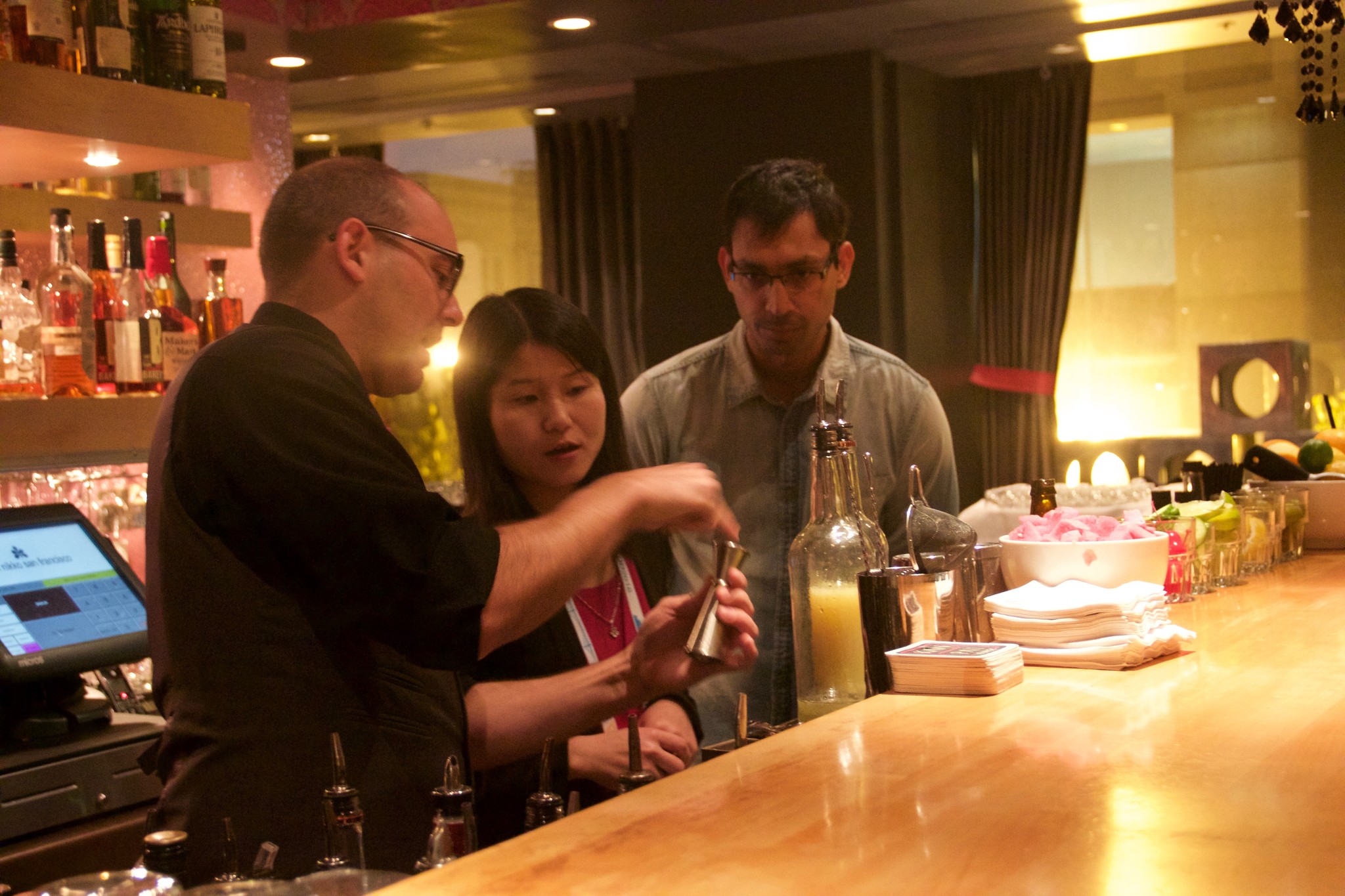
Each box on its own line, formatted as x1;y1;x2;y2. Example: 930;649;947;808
1247;478;1345;549
998;531;1170;588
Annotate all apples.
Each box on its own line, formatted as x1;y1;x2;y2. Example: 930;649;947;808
1147;491;1306;566
1325;446;1345;474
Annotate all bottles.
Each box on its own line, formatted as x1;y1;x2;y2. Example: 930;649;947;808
83;219;120;397
1029;476;1058;517
1182;461;1207;502
522;737;565;833
155;210;193;318
828;379;891;568
320;731;368;896
34;208;99;397
0;229;47;401
110;219;165;396
213;816;249;883
196;259;244;348
788;381;876;725
616;711;656;794
0;0;228;100
426;754;476;869
144;235;201;396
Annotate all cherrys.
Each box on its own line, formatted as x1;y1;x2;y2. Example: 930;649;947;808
1151;500;1193;596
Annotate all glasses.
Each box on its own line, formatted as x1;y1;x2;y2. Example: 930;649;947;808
727;245;835;293
328;224;463;301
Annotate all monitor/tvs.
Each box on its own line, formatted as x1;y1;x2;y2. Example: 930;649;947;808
0;502;149;677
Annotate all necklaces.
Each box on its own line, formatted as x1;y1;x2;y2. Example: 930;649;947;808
575;565;621;638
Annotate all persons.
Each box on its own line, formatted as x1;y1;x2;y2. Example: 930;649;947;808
455;287;706;849
146;160;757;881
621;153;963;727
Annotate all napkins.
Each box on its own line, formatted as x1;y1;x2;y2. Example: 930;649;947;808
980;581;1196;674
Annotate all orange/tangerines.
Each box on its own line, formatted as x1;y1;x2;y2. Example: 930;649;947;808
1253;439;1301;464
1297;438;1333;473
1314;429;1345;453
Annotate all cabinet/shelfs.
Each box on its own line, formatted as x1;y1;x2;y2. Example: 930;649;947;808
0;67;259;466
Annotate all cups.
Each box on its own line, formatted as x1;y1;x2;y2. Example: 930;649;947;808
1115;486;1309;604
12;866;412;896
855;564;982;697
893;543;1007;642
681;539;750;664
